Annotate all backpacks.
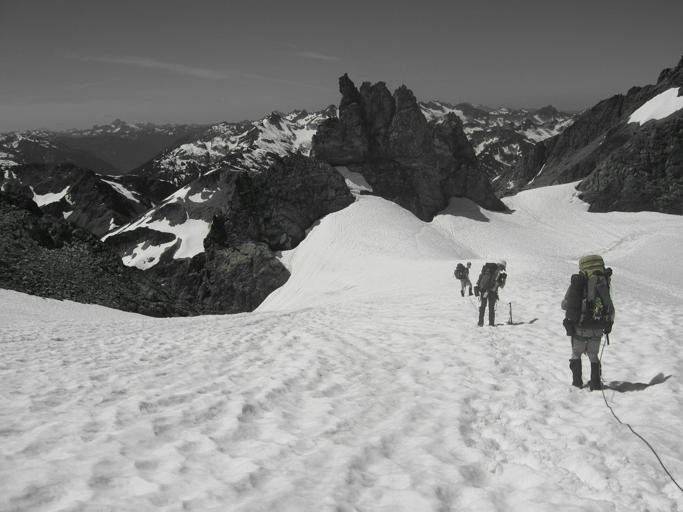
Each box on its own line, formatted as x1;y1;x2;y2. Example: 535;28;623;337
454;263;465;279
479;263;496;292
580;255;610;327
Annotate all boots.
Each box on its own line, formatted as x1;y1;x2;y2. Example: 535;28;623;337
588;362;602;390
461;289;465;297
489;308;495;326
477;306;485;327
468;286;474;296
568;358;584;387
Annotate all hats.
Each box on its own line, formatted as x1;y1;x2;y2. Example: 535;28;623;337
496;259;507;268
466;261;472;265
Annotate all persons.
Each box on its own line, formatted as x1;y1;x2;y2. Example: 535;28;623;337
474;261;506;327
562;255;614;390
452;263;472;296
